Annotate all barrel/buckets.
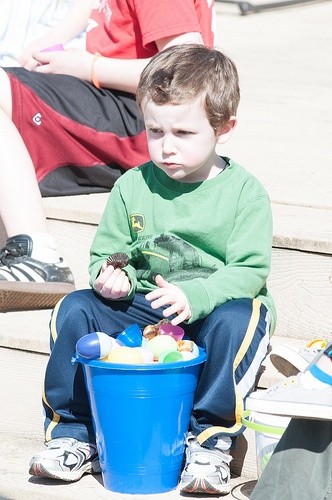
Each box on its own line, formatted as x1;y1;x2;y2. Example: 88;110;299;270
241;410;306;479
74;346;206;494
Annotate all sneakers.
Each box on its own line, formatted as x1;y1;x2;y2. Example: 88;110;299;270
270;339;329;378
0;234;78;311
179;431;233;495
29;435;104;482
245;344;332;421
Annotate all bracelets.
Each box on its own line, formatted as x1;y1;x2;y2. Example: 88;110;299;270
91;52;102;89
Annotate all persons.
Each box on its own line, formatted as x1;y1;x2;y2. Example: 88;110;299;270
0;0;215;313
28;43;332;500
